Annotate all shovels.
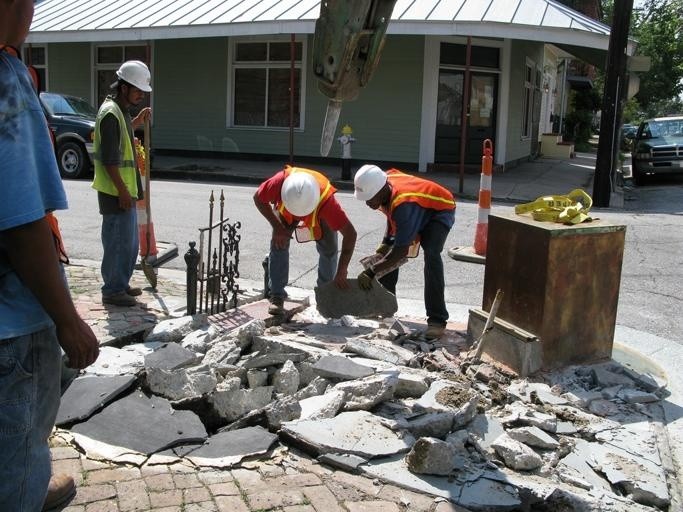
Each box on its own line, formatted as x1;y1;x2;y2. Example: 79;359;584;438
140;107;157;290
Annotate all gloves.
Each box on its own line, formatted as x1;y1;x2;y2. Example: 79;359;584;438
376;244;389;255
358;267;374;290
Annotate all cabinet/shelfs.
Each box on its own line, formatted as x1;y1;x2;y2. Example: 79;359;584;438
539;132;578;161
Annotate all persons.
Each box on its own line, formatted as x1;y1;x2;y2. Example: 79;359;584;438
352;162;455;339
89;60;155;305
251;163;358;315
0;2;98;511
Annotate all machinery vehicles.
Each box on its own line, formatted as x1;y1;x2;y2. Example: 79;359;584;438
310;0;396;156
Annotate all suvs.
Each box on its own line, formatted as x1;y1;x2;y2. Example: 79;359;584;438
37;91;97;178
626;116;682;185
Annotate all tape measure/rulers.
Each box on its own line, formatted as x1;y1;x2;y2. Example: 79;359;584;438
515;188;600;225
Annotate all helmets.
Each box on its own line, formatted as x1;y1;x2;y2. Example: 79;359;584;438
281;173;320;216
354;165;387;200
116;60;153;92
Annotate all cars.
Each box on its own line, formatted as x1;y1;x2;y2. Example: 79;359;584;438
621;125;639;147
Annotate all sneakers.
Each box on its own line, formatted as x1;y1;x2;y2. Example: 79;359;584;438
44;473;75;509
425;320;445;339
268;297;283;314
102;286;141;306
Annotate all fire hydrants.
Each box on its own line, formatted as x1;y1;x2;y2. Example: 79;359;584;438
337;124;356;180
